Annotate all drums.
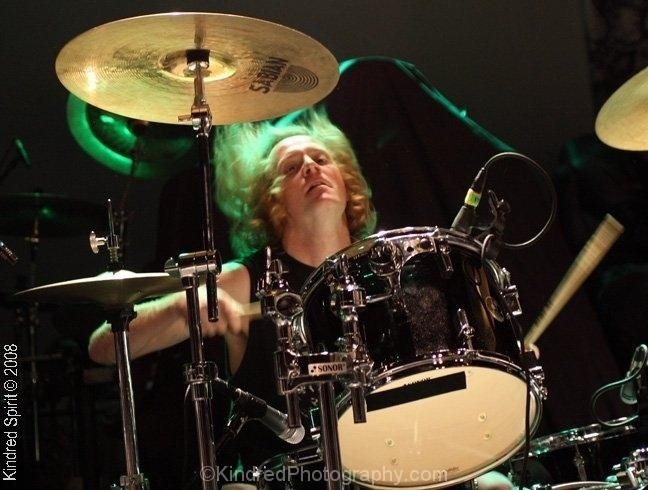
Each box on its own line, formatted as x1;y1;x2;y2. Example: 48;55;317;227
502;414;647;490
252;444;359;490
288;226;547;490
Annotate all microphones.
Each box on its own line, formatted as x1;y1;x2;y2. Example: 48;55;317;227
202;363;305;447
449;168;487;237
619;344;648;405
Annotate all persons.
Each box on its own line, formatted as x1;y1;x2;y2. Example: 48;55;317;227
87;103;517;489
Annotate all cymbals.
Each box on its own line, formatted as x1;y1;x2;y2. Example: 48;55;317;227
13;270;193;306
594;68;648;152
54;12;340;127
